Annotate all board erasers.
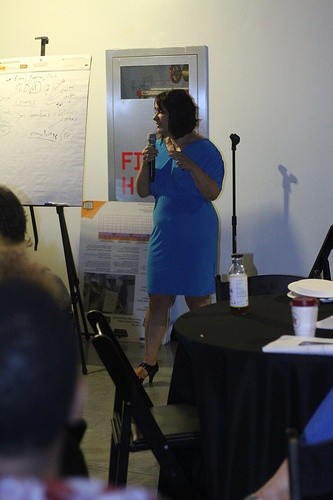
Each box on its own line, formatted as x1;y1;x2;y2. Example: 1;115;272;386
45;203;52;205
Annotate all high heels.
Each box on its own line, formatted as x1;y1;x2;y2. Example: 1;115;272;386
136;362;159;383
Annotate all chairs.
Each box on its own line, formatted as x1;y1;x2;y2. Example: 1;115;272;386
214;274;307;303
86;310;203;500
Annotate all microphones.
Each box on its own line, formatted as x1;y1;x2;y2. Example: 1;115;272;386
146;133;156;182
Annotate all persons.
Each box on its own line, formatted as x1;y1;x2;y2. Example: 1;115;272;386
83;276;128;315
0;248;155;500
0;184;69;294
135;89;224;384
241;389;333;500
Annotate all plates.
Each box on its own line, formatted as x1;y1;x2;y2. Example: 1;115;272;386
286;277;333;302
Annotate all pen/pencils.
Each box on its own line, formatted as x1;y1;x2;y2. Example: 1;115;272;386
173;144;184;170
48;202;68;205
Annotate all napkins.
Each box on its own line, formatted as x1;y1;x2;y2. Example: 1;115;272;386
316;315;333;330
262;335;333;356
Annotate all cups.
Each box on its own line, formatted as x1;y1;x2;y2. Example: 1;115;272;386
291;296;319;337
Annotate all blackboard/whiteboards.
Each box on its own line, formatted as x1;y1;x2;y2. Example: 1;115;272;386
0;55;92;207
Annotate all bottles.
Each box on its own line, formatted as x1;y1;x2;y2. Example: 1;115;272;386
228;253;250;315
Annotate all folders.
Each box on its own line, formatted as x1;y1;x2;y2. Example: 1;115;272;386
131;403;201;443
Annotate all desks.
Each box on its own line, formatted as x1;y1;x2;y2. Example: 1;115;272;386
156;292;333;500
